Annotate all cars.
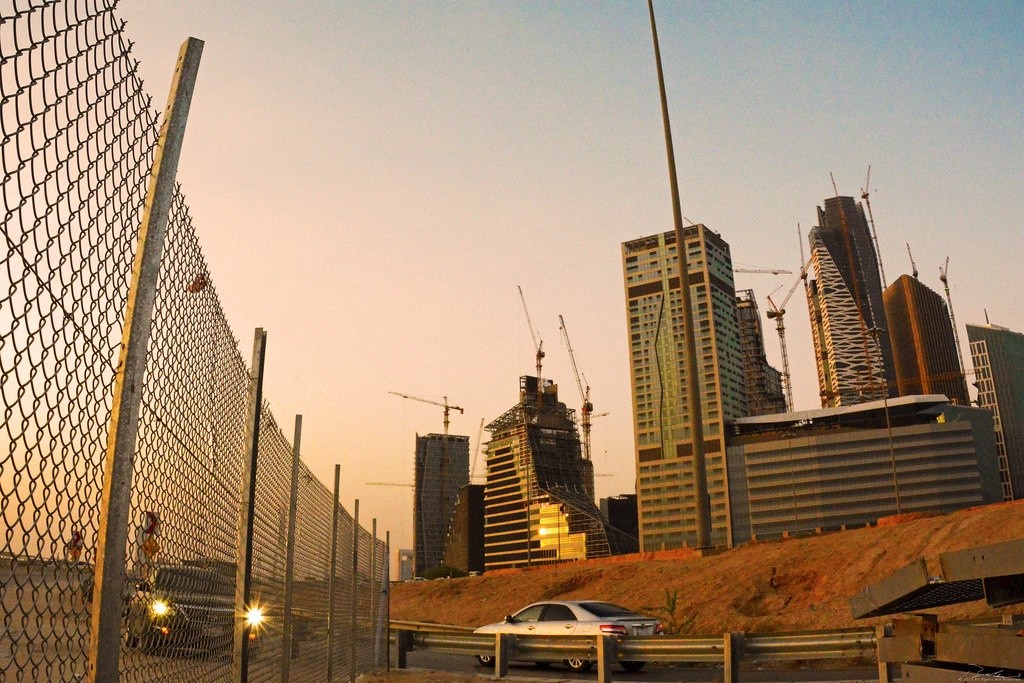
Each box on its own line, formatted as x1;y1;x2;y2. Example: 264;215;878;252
469;599;665;673
74;558;307;657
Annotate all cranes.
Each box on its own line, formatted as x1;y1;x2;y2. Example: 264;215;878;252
761;163;971;413
517;283;548;441
386;389;464;579
558;314;597;459
